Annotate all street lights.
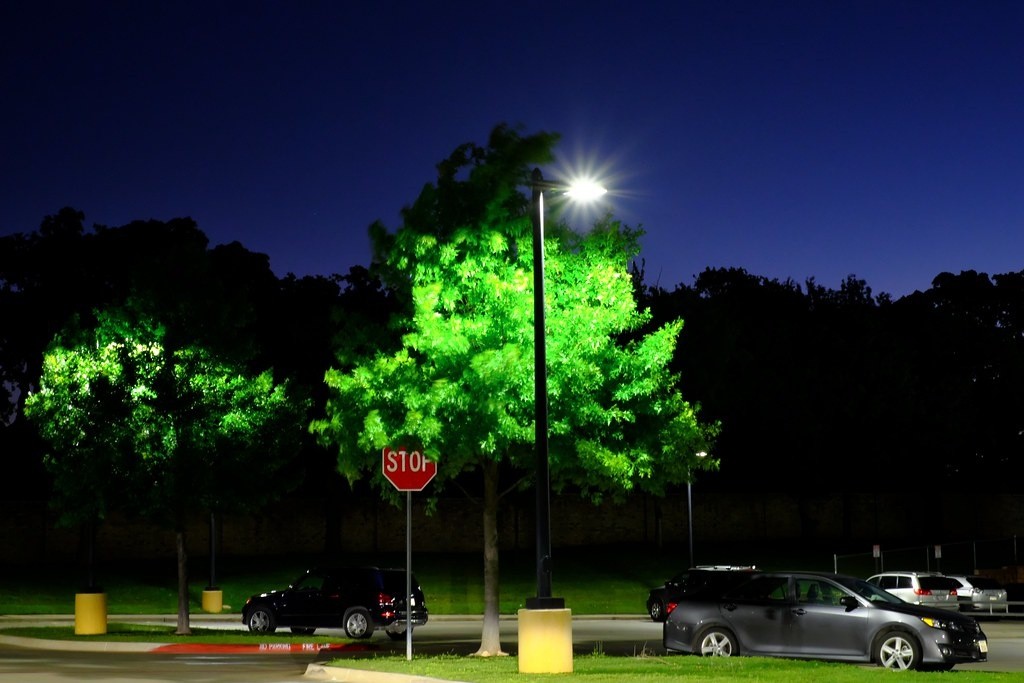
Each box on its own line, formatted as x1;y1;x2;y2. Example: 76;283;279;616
517;164;615;673
686;451;708;566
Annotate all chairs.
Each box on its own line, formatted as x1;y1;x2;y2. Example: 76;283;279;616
807;584;820;603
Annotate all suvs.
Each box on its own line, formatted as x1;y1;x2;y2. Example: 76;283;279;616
646;565;760;621
242;563;429;642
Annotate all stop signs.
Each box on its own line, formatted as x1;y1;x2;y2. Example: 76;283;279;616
381;438;438;491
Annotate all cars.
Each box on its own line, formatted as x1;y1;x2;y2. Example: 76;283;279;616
663;570;988;672
951;574;1007;612
862;572;961;613
